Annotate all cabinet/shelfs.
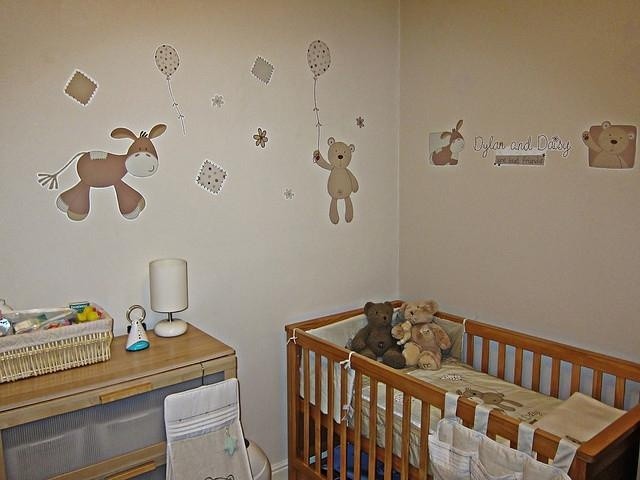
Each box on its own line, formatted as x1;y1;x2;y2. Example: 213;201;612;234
0;322;238;480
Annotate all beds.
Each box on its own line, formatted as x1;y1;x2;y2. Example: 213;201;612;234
285;300;640;480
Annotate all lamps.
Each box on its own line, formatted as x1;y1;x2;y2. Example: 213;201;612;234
150;258;188;337
